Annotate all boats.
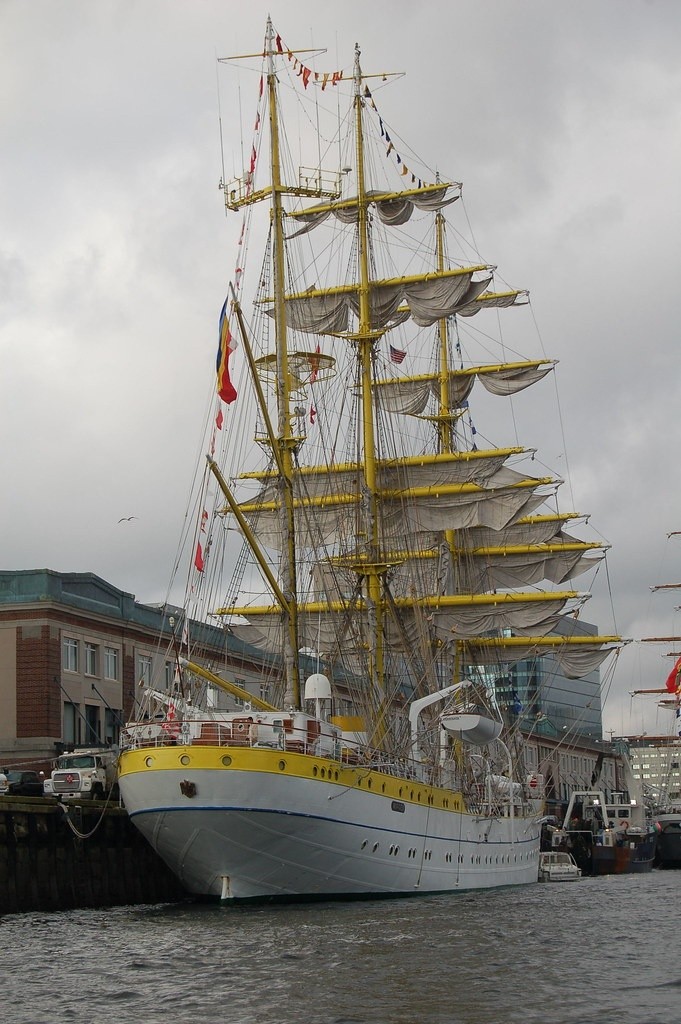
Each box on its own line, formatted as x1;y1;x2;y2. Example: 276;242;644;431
551;788;659;873
439;712;502;746
537;850;582;883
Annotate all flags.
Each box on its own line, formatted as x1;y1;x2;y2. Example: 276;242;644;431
276;34;344;91
390;345;407;364
236;41;266;291
160;407;223;737
310;405;315;425
365;86;435;189
310;346;320;385
666;657;681;736
216;296;237;405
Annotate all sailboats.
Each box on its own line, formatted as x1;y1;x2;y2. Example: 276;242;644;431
117;11;632;899
629;530;681;868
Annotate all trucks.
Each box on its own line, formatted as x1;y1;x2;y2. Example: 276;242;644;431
43;747;119;803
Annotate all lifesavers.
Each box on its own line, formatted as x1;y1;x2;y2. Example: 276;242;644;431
620;821;629;829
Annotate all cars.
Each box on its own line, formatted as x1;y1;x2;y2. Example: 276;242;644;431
0;770;43;797
